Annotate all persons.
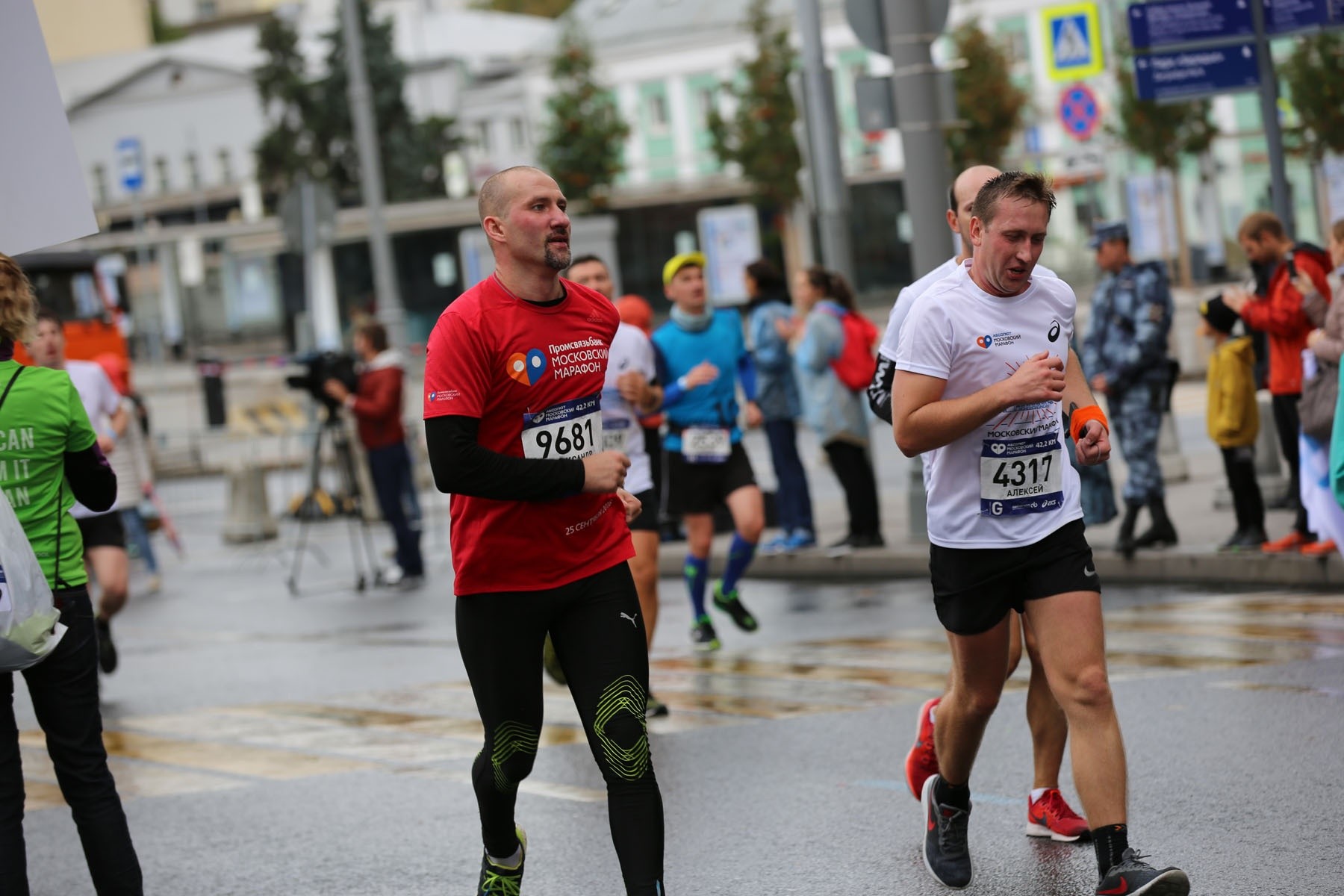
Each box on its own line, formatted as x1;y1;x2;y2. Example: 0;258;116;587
323;318;426;594
891;169;1190;896
563;249;817;719
24;310;162;677
424;164;665;896
1196;295;1270;552
0;251;146;896
1079;221;1176;555
774;267;885;557
1222;210;1344;558
865;163;1094;843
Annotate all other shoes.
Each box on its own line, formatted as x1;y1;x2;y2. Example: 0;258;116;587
1300;537;1337;555
543;633;570;686
1221;525;1269;550
645;693;669;718
94;614;118;675
376;564;423;586
711;580;757;632
690;615;720;652
825;536;885;559
763;528;816;552
1261;530;1312;554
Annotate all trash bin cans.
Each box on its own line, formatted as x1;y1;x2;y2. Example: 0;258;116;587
196;357;225;424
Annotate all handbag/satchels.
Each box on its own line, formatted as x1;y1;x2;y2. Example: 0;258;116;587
1296;355;1339;436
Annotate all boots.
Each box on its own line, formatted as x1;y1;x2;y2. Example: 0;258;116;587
1135;492;1178;548
1115;498;1141;554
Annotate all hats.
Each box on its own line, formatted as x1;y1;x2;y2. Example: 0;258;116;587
662;253;705;285
1197;293;1248;339
1086;222;1128;247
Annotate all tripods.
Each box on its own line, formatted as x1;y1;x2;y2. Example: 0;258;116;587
287;410;384;592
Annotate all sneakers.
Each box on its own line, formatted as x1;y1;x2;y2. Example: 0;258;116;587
1095;848;1191;896
905;696;941;803
476;823;528;896
1025;787;1091;843
922;773;974;890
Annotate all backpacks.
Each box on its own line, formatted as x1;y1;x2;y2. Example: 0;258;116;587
0;365;69;672
813;305;880;390
